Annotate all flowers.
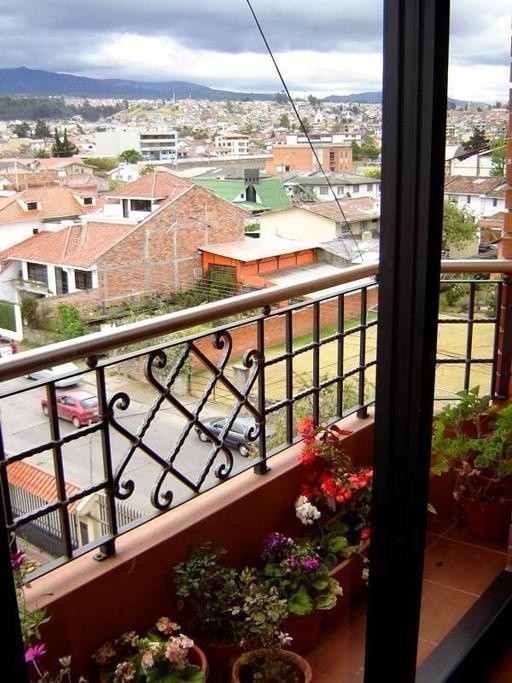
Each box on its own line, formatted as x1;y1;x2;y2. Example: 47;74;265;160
295;417;373;586
91;616;207;683
257;532;343;616
222;586;291;678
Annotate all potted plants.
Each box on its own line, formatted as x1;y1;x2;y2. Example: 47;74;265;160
429;386;512;540
171;543;239;673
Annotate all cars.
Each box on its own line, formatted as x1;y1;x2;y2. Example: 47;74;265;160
41;390;100;429
192;414;277;457
0;333;17;358
23;361;86;388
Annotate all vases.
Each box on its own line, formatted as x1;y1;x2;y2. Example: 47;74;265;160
279;610;323;649
99;643;209;682
233;648;312;683
327;510;369;597
